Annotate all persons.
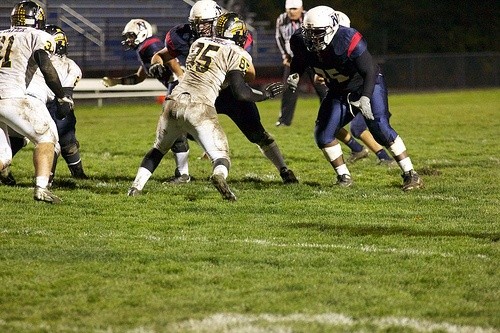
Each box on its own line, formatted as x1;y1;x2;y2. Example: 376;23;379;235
128;13;290;200
271;0;333;128
2;23;89;181
148;0;300;186
302;5;426;190
102;14;215;184
306;11;402;166
0;0;74;206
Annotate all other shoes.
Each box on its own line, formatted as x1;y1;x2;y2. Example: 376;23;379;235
280;166;298;184
33;187;63;203
400;168;424;192
210;174;236;201
170;174;191;184
0;171;16;186
347;144;369;164
376;158;396;168
331;174;357;189
275;121;289;127
127;187;142;197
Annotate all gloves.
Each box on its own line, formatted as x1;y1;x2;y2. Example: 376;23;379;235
57;94;75;112
350;96;375;121
264;81;286;98
287;73;300;91
102;76;117;87
148;62;164;79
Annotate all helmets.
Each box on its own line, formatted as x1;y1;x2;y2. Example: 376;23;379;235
120;19;153;53
10;0;47;30
43;24;68;57
214;11;248;49
300;5;340;54
188;0;223;37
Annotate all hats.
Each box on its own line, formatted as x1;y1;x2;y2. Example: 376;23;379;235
285;0;303;10
335;11;350;28
